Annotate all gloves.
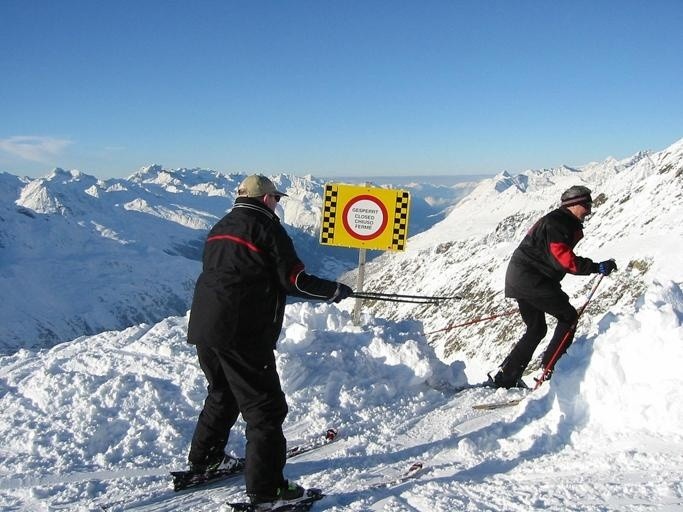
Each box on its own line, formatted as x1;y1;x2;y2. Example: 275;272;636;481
596;260;616;276
327;284;354;304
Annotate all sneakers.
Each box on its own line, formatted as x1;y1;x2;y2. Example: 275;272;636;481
495;371;526;388
249;479;303;504
189;454;244;473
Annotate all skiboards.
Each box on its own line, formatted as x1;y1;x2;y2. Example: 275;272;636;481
168;422;423;511
444;380;526;410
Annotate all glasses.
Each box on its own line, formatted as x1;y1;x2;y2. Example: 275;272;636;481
269;196;282;202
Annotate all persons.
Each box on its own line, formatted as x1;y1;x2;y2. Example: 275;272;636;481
187;176;353;505
495;185;617;389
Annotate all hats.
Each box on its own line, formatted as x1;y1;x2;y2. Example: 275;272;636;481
562;186;591;206
237;175;287;196
